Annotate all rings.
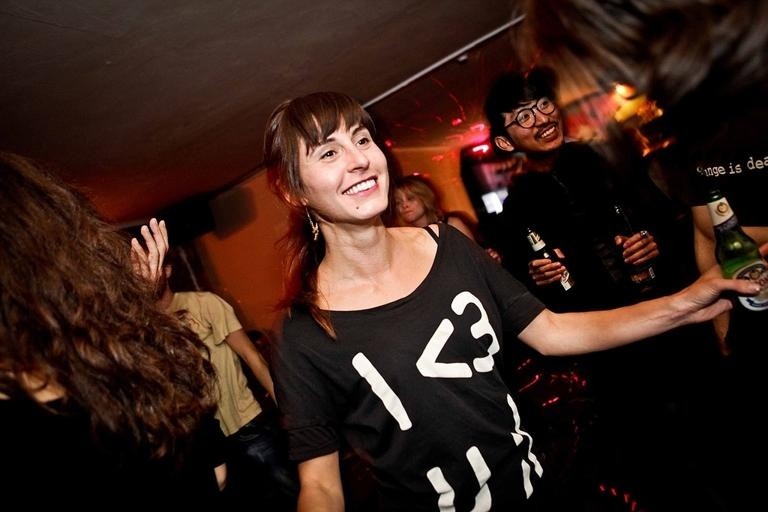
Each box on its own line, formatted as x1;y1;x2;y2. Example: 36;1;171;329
640;230;649;238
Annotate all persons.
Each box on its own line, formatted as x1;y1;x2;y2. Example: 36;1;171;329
393;174;503;264
153;253;297;511
683;157;767;348
0;146;237;511
260;90;768;512
482;65;694;312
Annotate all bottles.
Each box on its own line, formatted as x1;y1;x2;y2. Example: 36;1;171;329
703;185;768;326
522;226;580;299
610;202;665;301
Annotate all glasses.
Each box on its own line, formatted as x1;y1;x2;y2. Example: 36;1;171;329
493;94;557;135
